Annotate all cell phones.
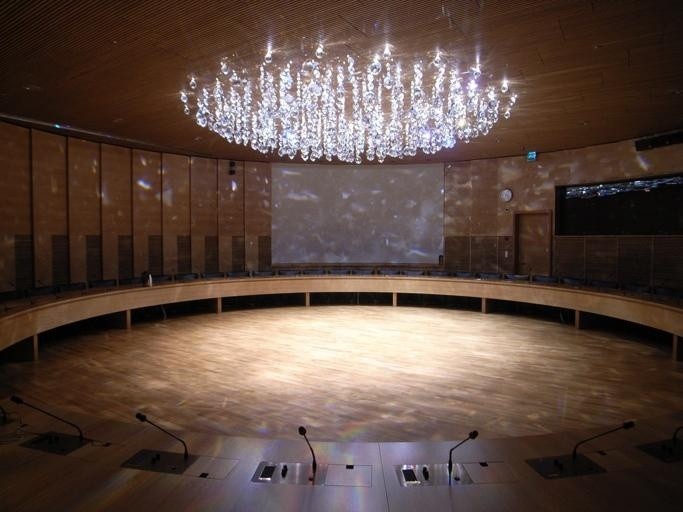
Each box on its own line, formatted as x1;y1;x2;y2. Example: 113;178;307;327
258;465;276;480
401;468;419;485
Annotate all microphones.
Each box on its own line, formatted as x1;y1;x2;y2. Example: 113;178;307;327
135;412;189;460
37;279;64;299
553;421;635;473
448;430;478;472
8;280;35;305
297;426;317;481
9;394;84;447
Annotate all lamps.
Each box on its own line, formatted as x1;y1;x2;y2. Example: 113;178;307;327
177;6;518;166
